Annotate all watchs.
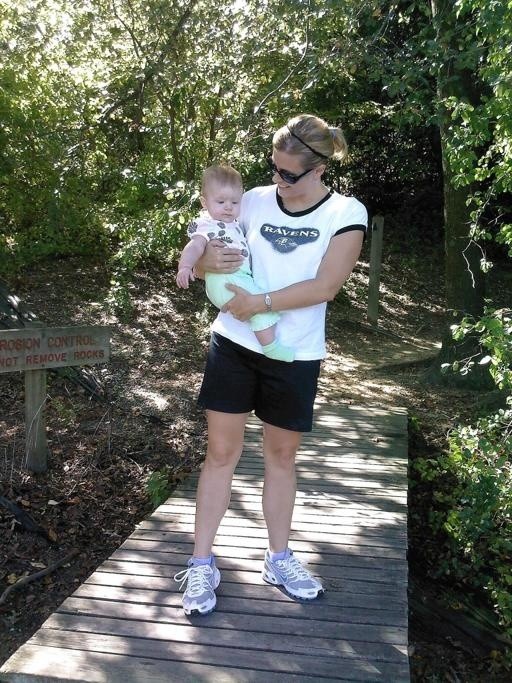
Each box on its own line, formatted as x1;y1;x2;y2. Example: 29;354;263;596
264;293;271;312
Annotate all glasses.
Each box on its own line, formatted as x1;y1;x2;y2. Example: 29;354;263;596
266;150;313;184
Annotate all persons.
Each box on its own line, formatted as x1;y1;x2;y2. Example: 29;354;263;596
175;114;369;617
176;166;296;362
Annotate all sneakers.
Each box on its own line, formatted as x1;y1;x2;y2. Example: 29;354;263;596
181;555;221;616
262;550;325;600
